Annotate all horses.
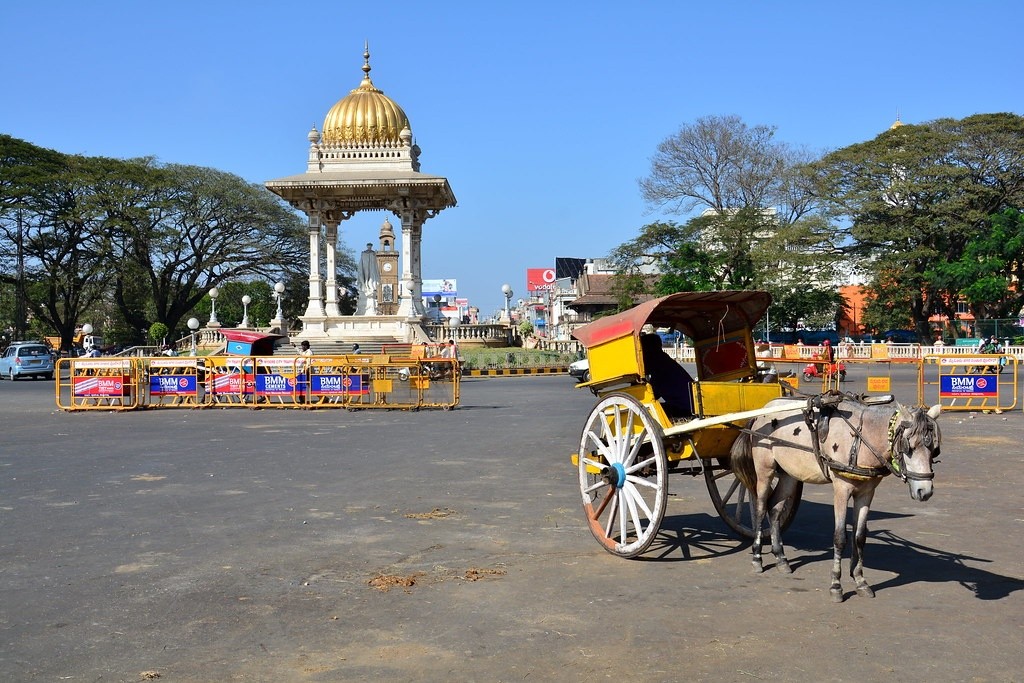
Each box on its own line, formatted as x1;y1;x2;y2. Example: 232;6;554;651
731;389;943;604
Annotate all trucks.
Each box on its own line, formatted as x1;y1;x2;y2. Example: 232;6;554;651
43;329;104;358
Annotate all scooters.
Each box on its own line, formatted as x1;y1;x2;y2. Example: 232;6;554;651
397;357;464;382
801;351;847;383
964;348;1005;374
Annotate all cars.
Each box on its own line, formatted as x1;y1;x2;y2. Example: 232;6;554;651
0;344;54;381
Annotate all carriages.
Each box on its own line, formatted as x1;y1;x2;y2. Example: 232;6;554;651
565;290;944;603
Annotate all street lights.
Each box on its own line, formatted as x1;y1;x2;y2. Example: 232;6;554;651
236;294;253;328
405;280;418;318
205;288;223;328
448;317;461;345
433;293;443;325
274;282;287;319
502;284;513;316
187;317;200;356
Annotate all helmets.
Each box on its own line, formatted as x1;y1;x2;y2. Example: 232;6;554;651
353;344;359;349
824;340;830;345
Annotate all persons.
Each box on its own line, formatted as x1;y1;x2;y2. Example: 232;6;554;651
357;242;380;292
817;340;833;373
979;335;1002;354
421;340;457;378
89;345;101;358
349;343;363;373
159;341;183;373
290;340;329;404
640;333;696;416
756;344;776;383
885;336;894;343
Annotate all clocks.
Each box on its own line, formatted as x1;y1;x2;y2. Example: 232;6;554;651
382;262;392;272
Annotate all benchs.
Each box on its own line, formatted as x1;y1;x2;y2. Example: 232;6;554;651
642;334;693;417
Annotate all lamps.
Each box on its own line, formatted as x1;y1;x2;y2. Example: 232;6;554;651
82;322;92;351
208;288;220;326
186;317;200;356
406;280;422;314
449;317;463;358
273;281;286;321
500;282;515;320
241;295;251;324
433;293;442;323
